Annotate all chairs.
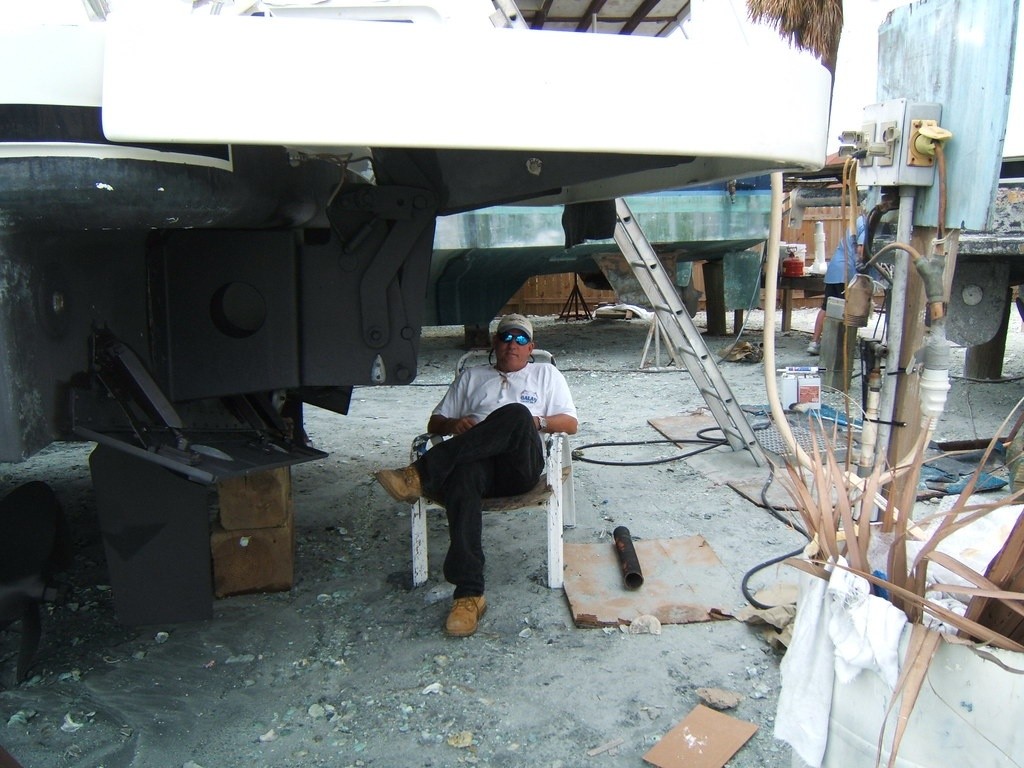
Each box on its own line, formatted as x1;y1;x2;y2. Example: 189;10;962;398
410;349;574;588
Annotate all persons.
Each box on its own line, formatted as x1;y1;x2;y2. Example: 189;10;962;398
807;217;865;354
377;313;578;638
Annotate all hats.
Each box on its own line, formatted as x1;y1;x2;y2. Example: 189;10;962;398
497;314;533;342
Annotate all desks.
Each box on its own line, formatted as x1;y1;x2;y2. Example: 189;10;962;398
736;274;827;340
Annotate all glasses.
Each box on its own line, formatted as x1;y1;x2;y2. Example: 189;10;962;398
498;332;530;346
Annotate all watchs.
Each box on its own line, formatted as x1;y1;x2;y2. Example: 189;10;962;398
539;417;547;431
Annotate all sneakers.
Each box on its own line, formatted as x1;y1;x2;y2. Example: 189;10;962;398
377;467;423;504
446;594;487;636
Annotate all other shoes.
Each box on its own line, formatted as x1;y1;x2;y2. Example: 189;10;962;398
806;338;822;354
